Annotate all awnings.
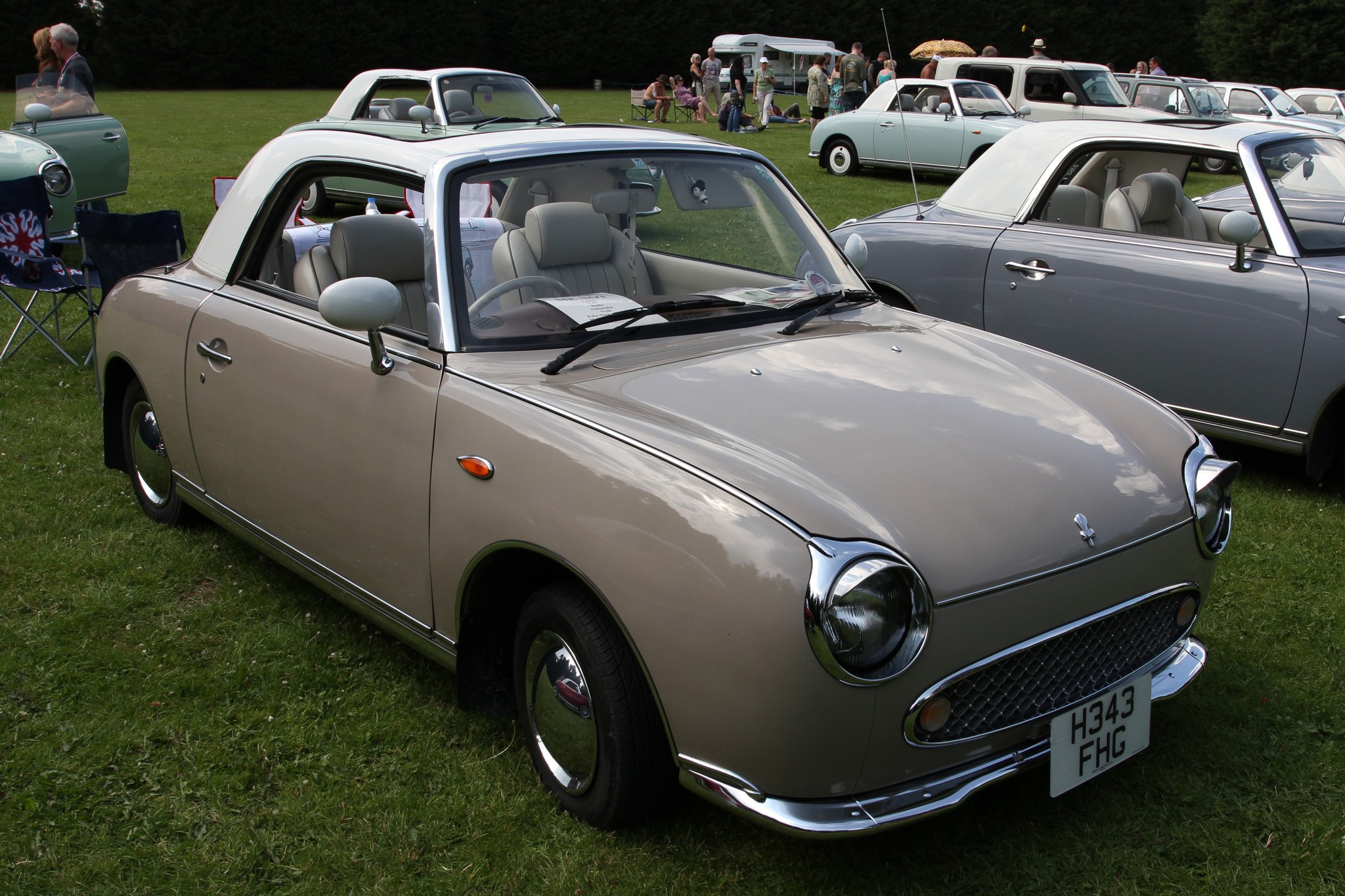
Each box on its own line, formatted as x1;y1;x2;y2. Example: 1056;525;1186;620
766;44;848;96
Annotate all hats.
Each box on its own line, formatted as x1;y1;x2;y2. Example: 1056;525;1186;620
760;57;768;63
933;54;941;62
656;75;670;85
1030;39;1046;48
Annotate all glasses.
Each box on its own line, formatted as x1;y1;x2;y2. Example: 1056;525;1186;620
678;79;684;82
694;58;700;59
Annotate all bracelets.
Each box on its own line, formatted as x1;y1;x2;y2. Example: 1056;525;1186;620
739;93;742;95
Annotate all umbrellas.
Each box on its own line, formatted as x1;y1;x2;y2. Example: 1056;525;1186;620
909;38;976;61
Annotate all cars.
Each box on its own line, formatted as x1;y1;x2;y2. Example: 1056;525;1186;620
97;129;1239;842
0;71;131;243
793;118;1344;487
807;77;1037;177
1083;73;1344;141
280;66;565;218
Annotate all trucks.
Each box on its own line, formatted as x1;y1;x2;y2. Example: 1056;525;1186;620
711;33;848;93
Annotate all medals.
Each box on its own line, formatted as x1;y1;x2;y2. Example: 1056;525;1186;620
765;76;768;78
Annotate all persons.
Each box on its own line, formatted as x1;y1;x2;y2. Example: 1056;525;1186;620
35;23;109;213
859;52;870;96
920;55;942;79
1027;39;1055;92
1137;61;1148;104
1129;69;1137;75
868;51;889;94
690;53;704;121
726;56;747;133
642;75;674;123
718;92;767;131
769;99;806;124
839;43;867;112
669;75;718;123
876;60;897;87
977;46;997;58
752;57;776;128
807;55;829;133
773;103;810;124
829;54;846;116
1147;57;1168;101
701;47;722;113
1105;63;1115;73
935;91;955;115
814;53;832;92
32;28;60;97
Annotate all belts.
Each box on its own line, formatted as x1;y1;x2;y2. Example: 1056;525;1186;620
692;81;702;83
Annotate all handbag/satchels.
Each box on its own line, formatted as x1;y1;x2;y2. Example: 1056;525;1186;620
691;87;697;97
730;92;745;105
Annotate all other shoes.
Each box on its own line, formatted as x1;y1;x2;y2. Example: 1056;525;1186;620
758;125;767;131
766;126;769;129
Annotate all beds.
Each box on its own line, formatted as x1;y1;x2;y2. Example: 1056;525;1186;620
1101;173;1207;244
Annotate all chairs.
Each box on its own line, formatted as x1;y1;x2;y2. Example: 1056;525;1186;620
1;175;106;368
922;95;941;115
1047;185;1102;230
630;89;660;122
893;93;921;112
77;205;189;409
293;212;462;335
435;88;485;122
378;98;434;123
491;201;653;313
672;89;704;122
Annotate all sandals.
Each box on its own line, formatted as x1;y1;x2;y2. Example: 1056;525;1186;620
660;118;669;123
654;119;661;123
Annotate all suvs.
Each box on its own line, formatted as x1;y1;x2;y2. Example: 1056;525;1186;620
933;57;1182;125
